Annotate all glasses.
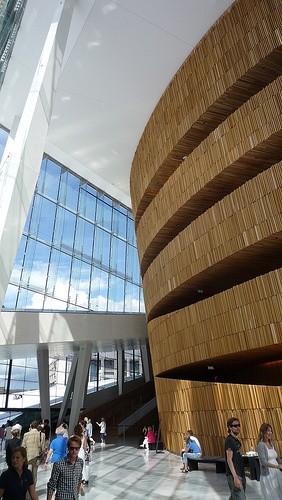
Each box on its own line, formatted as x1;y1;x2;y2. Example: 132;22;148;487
69;446;80;451
229;423;241;428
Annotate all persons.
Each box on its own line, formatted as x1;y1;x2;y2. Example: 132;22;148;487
0;445;40;500
0;417;107;486
224;418;247;500
179;430;202;474
256;423;282;500
140;427;156;450
46;435;84;500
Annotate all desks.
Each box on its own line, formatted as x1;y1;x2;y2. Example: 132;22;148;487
242;455;259;479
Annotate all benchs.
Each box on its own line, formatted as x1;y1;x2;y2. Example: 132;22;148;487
188;456;226;473
149;442;163;450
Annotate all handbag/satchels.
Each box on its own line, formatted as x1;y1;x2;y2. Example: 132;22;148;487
38;432;44;461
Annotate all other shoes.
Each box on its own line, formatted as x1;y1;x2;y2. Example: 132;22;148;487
181;470;188;473
